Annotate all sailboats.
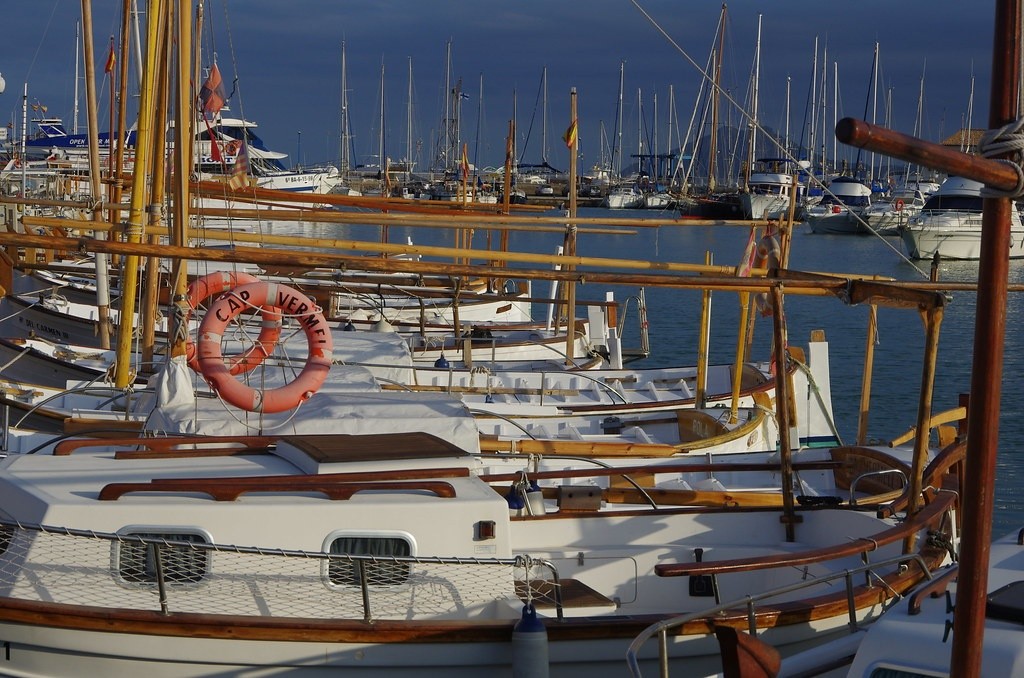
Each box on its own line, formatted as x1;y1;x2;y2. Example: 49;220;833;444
0;0;1024;678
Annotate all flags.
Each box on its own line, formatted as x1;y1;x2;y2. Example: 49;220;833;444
461;144;470;177
104;36;118;74
198;65;226;113
228;139;249;192
561;118;580;149
734;226;756;278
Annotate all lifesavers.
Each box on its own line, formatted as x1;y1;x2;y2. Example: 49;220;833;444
896;199;904;211
14;158;22;169
218;144;224;152
198;280;335;415
226;143;236;154
167;271;283;376
